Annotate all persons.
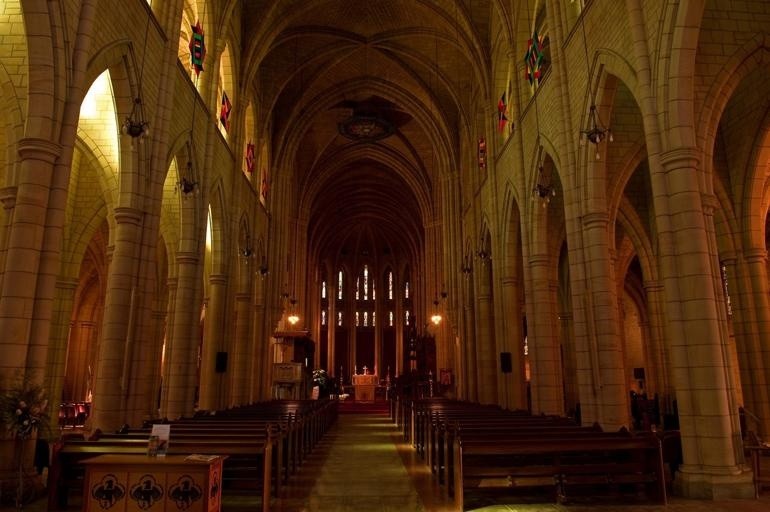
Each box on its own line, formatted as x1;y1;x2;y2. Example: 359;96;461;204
629;390;682;476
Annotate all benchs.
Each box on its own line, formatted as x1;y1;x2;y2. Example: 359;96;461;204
48;397;340;512
386;391;671;504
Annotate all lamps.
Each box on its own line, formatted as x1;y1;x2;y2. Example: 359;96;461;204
170;1;210;205
460;238;494;281
524;1;565;210
573;1;614;163
233;213;291;300
121;1;156;154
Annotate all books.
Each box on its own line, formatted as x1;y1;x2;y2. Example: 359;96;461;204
185;454;219;463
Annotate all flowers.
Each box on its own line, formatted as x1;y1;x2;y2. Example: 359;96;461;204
2;359;51;436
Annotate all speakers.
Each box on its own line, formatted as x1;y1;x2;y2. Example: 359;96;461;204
216;352;228;373
500;352;512;373
